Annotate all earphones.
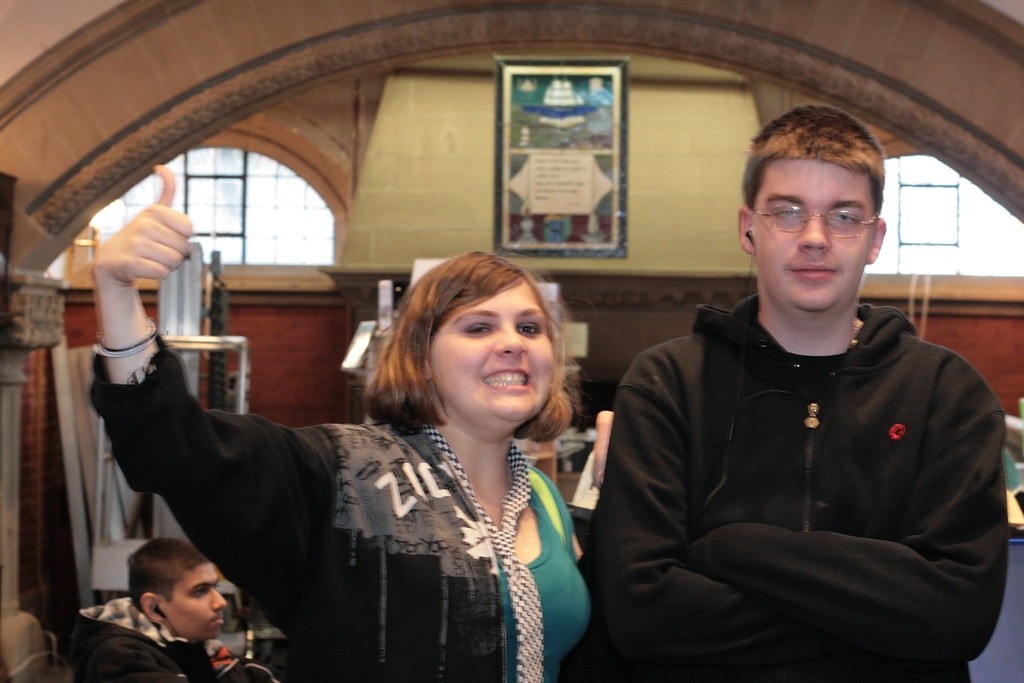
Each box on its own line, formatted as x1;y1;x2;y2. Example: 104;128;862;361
746;230;755;248
149;604;167;619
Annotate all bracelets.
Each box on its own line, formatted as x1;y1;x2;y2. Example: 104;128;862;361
98;321;157;357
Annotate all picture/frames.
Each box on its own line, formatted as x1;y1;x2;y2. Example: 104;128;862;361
492;54;629;260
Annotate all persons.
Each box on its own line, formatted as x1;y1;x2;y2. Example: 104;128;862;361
568;106;1009;683
72;538;283;683
90;166;613;683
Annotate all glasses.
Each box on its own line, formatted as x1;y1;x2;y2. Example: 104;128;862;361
754;206;879;237
